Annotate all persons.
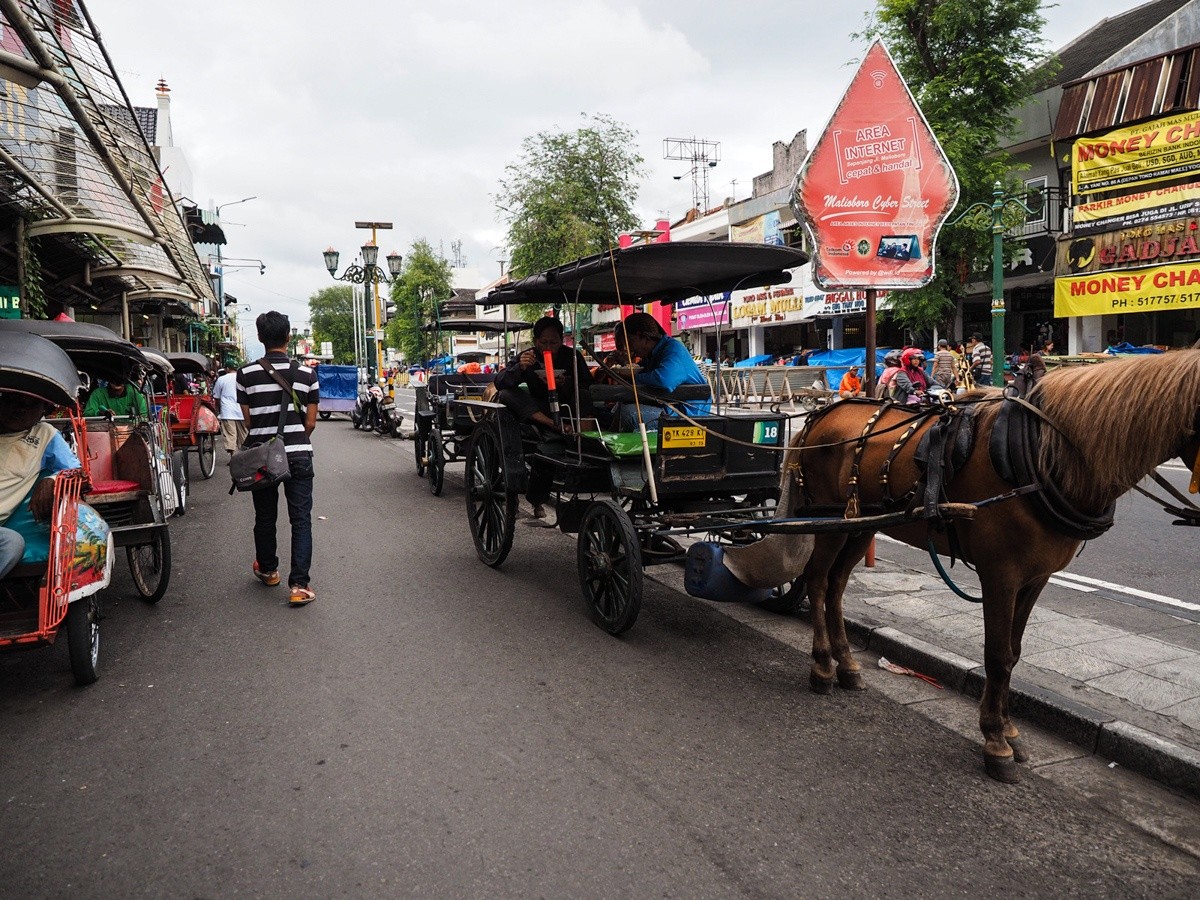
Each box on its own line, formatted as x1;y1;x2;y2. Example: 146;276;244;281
874;348;902;403
84;384;145;418
494;316;591;432
930;339;971;388
1;381;81;577
620;312;712;432
153;367;221;411
388;367;393;376
890;347;945;405
838;365;861;398
1038;340;1054;357
970;332;991;385
238;311;318;604
213;361;248;464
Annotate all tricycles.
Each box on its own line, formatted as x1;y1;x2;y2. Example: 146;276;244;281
0;318;219;687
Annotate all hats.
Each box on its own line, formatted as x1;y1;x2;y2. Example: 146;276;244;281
625;313;666;338
969;333;983;340
937;339;947;345
225;360;239;368
850;366;859;371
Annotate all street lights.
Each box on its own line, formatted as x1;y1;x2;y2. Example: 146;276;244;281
215;194;258;312
323;241;403;391
206;254;267;362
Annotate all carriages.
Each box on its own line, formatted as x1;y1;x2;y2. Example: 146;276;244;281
466;241;1200;783
414;317;574;519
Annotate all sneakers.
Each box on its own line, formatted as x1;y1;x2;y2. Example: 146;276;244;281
288;584;316;604
253;560;281;587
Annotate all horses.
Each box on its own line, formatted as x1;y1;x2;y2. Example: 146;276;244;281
777;344;1200;783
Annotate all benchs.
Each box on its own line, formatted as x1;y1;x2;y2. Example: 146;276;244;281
429;373;500;429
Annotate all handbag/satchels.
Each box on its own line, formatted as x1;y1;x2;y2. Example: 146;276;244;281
227;433;291;495
971;368;982;380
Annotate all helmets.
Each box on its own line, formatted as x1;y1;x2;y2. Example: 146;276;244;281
884;349;904;367
902;348;924;368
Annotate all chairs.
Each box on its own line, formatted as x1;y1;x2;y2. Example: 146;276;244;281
154;396;197;430
0;468;56;576
70;430;142;503
